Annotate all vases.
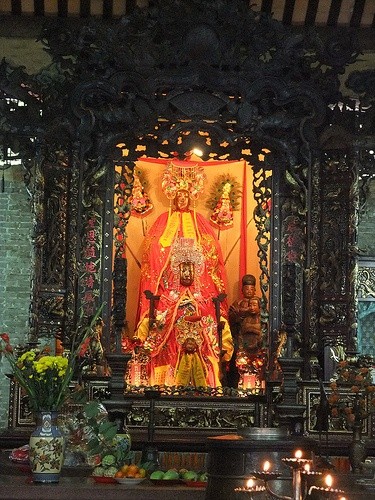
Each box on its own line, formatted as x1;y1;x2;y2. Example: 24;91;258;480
348;426;369;474
29;410;65;485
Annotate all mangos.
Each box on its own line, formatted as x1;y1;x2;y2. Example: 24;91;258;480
184;471;208;482
150;470;179;480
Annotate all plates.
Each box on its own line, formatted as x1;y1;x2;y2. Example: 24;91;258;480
149;477;180;486
185;479;208;487
89;475;116;484
114;477;146;486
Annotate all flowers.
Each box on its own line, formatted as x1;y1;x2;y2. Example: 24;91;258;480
327;323;375;428
0;301;107;411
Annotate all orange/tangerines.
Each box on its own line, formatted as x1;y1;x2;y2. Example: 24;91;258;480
115;464;146;478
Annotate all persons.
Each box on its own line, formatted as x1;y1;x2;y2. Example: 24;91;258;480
229;274;262;342
240;297;263;352
134;161;230;318
143;240;235;386
